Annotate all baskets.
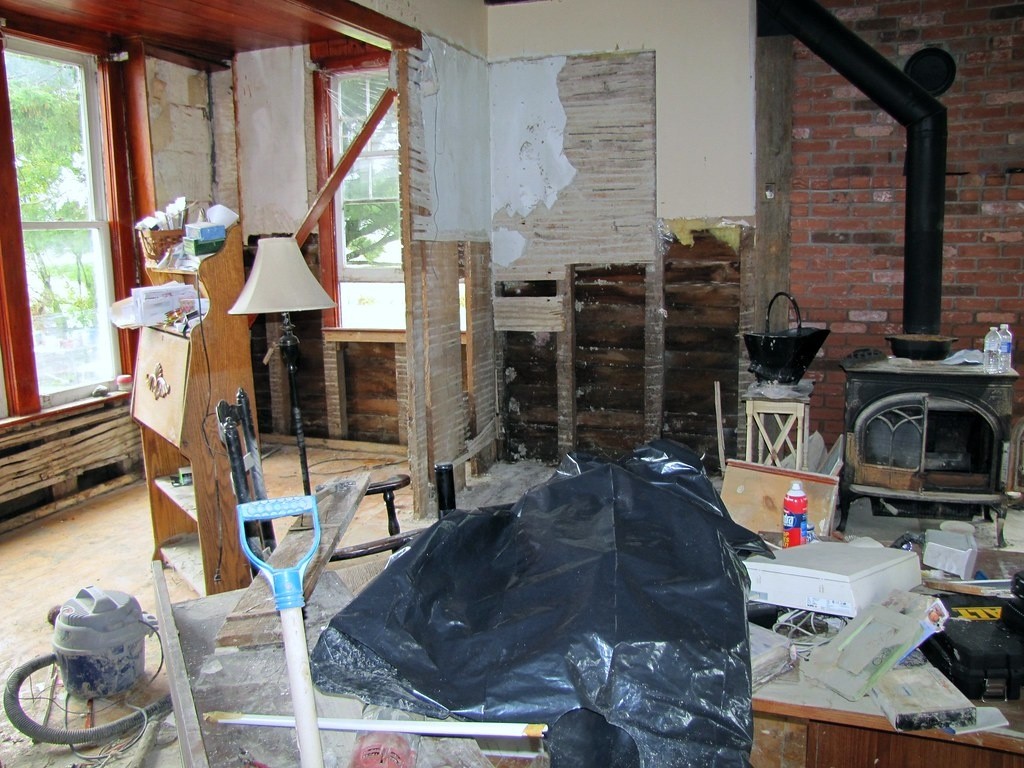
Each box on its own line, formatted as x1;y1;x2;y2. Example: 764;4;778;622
137;229;186;265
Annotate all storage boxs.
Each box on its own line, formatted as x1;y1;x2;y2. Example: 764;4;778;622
186;222;227;241
182;237;226;255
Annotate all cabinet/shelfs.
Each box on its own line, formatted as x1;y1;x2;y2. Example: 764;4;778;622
748;540;1024;768
128;225;259;602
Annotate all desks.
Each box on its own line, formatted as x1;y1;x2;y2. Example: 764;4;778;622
740;383;810;471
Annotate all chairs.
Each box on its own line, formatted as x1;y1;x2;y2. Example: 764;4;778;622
216;387;426;595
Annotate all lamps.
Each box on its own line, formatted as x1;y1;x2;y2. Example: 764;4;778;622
226;238;340;493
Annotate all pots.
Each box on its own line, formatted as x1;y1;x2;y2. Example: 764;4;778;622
743;294;831;386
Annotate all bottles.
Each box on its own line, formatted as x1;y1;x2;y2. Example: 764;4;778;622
983;327;1000;374
784;480;807;547
998;324;1012;372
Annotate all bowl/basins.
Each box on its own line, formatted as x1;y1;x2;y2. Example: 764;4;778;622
885;334;959;360
